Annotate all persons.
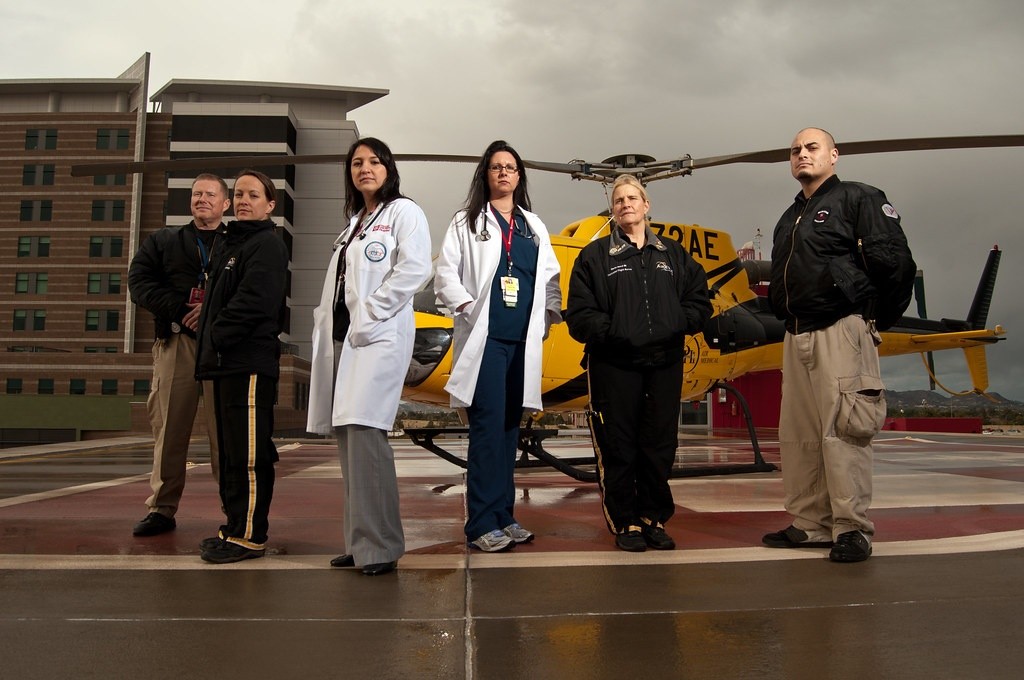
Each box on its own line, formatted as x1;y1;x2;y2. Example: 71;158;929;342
194;168;289;563
432;141;563;554
127;174;231;536
566;174;713;551
305;136;435;576
761;126;918;562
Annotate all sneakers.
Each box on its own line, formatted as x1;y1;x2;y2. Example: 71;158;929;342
762;525;834;548
199;536;265;563
829;530;872;561
466;523;534;552
133;512;176;536
615;527;675;552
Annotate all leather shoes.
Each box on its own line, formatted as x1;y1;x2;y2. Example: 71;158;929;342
362;560;397;576
330;555;356;566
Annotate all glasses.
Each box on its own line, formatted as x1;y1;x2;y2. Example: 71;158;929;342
487;163;519;174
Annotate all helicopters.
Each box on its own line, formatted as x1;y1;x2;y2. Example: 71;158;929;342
70;134;1024;484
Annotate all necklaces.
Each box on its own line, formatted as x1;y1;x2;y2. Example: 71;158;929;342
490;198;516;214
